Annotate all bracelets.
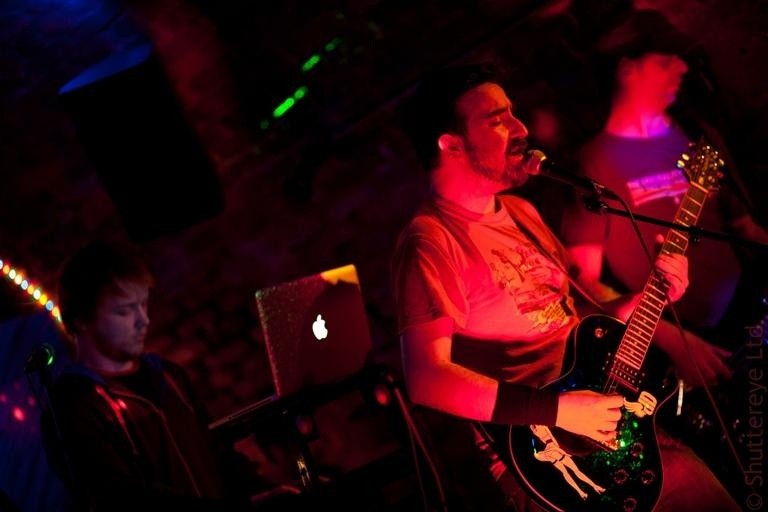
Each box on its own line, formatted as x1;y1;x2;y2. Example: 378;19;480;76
492;381;559;430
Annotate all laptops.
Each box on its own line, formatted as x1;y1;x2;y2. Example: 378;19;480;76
206;264;372;431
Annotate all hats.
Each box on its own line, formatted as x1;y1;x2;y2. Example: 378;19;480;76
595;8;698;65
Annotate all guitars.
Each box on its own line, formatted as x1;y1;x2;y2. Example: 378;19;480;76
665;233;765;445
505;140;726;512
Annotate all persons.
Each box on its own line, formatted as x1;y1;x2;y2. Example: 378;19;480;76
559;9;767;386
40;242;332;512
529;424;608;500
389;79;743;512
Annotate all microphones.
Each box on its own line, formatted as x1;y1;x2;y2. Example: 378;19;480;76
521;149;619;200
22;341;58;374
